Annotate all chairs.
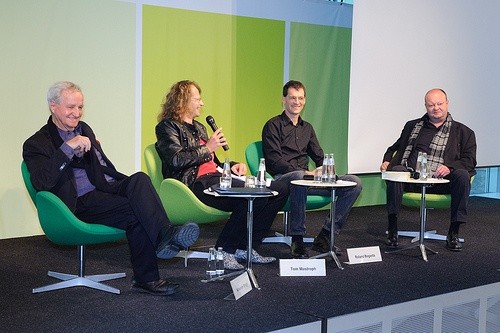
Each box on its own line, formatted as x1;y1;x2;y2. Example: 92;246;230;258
22;160;128;295
143;145;234;260
245;141;333;250
397;175;474;244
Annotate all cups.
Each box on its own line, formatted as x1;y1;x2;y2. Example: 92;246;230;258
219;177;232;189
313;169;322;183
245;176;255;188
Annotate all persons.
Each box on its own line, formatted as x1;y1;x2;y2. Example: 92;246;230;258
154;79;276;270
262;80;363;259
22;81;199;296
379;87;477;252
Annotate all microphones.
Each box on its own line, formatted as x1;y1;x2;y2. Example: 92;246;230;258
206;115;230;151
303;175;338;181
412;172;420;179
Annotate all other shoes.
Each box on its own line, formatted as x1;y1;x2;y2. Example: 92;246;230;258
235;249;275;263
222;251;245;270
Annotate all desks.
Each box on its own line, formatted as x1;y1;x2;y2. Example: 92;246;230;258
381;172;451;263
202;186;279;290
291;178;356;270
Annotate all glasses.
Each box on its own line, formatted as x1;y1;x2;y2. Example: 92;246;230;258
188;97;202;102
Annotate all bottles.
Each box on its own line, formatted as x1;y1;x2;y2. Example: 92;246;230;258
207;248;217;282
415;151;422;174
257;158;267;188
420;153;428;181
222;158;231;178
216;248;225;281
322;154;329;183
327;154;336;183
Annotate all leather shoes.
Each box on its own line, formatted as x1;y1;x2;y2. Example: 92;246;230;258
385;231;398;248
130;277;180;296
156;222;199;259
446;234;462;251
290;241;309;259
312;237;342;255
